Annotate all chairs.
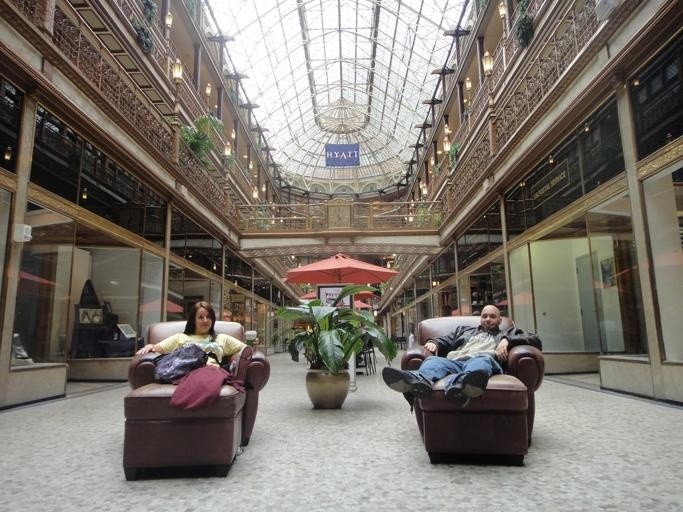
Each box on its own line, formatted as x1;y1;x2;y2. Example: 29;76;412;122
356;343;378;376
127;320;271;447
401;315;545;447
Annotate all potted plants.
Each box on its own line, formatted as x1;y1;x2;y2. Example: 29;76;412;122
267;284;396;410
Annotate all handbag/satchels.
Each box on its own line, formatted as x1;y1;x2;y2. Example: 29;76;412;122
152;345;207;383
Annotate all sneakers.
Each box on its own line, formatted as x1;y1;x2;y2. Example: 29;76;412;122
445;369;489;406
382;367;432;399
233;345;252;383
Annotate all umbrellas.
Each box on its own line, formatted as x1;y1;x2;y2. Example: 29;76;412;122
284;253;398;284
298;291;382;299
3;265;60;294
354;300;370;309
140;298;183;312
451;252;683;315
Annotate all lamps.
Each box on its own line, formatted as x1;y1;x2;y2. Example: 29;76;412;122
482;50;494;113
171;57;183;116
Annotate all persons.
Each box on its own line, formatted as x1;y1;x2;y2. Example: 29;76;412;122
382;305;542;403
135;301;252;380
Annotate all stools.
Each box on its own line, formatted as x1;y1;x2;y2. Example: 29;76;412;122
122;381;247;481
418;374;529;465
